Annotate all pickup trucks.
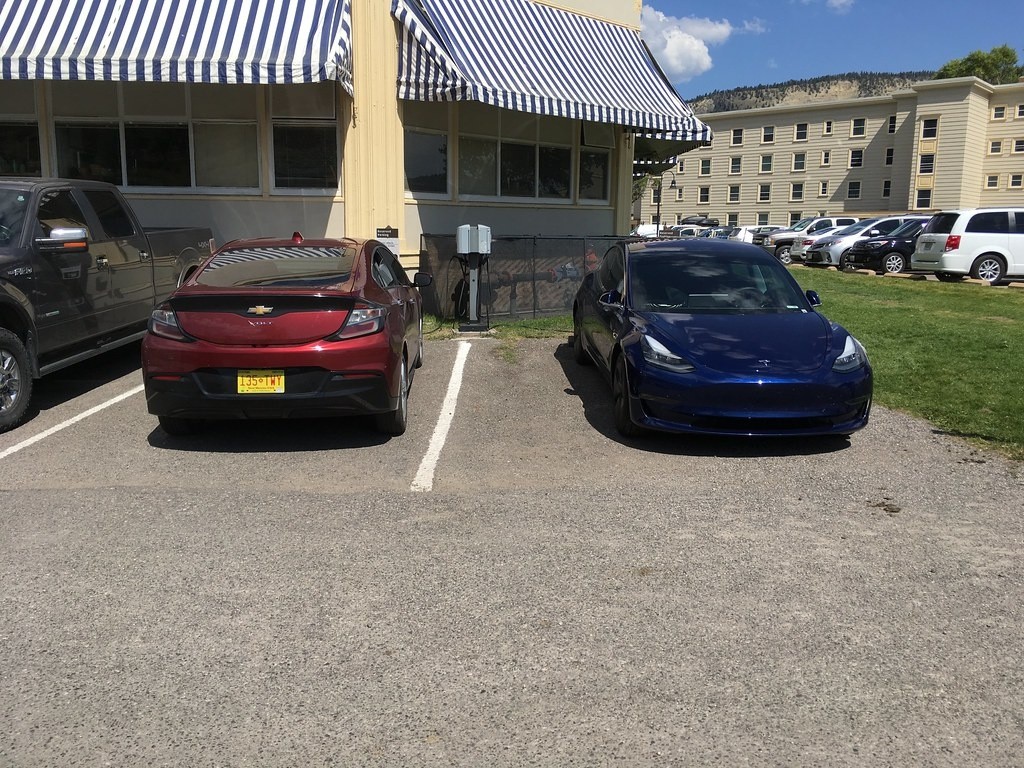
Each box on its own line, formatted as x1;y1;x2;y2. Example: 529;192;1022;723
0;176;217;434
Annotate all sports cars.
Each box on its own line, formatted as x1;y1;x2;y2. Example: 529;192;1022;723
572;236;874;439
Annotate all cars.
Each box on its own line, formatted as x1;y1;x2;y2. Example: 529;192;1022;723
140;230;432;436
847;217;929;275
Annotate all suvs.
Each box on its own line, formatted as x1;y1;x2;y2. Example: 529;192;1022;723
628;214;930;274
910;205;1024;286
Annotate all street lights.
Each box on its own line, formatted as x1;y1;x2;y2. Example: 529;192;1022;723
656;170;678;236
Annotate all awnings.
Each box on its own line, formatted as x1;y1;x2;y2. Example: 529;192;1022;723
391;0;715;180
0;0;354;101
